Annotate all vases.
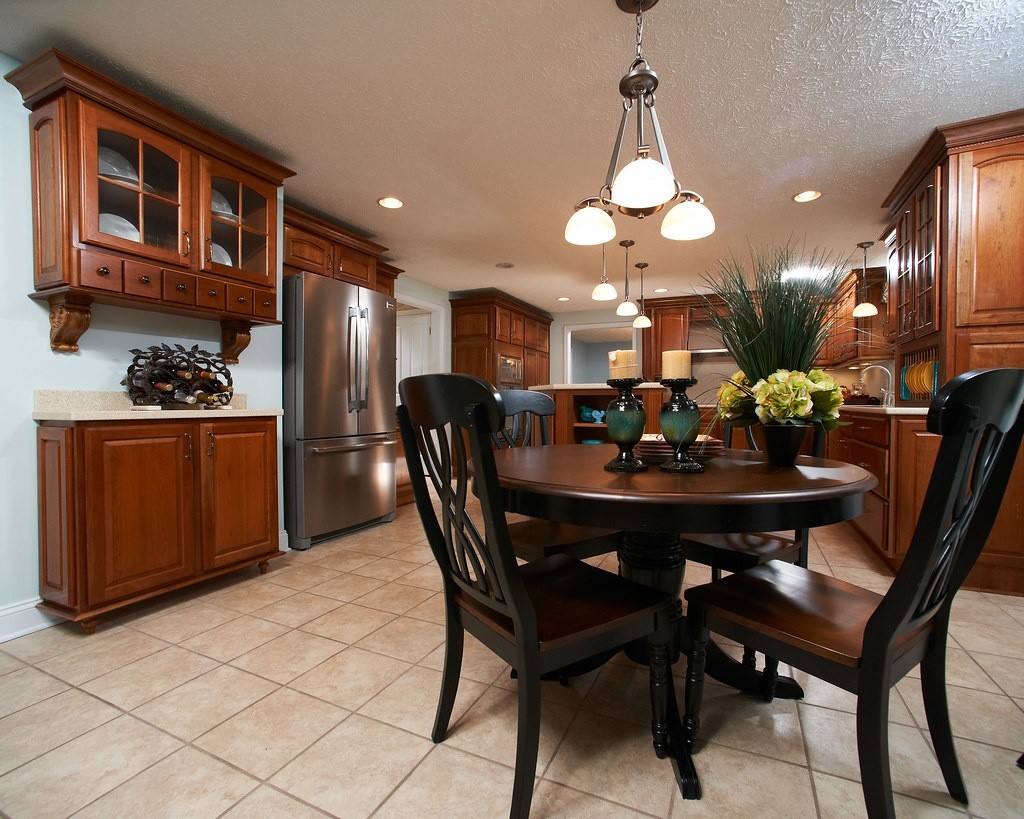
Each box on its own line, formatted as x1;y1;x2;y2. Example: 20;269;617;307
749;418;814;468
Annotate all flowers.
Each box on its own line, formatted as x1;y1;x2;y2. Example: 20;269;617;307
677;230;898;459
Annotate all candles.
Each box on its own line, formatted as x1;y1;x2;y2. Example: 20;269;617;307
608;350;638;379
662;350;691;380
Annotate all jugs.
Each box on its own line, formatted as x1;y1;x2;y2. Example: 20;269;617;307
850;383;869;404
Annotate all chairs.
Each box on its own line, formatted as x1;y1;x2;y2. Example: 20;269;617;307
467;390;623;687
683;367;1024;819
680;419;825;669
396;373;668;818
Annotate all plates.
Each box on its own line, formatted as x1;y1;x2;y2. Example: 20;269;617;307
633;433;727;464
212;242;233;267
905;361;935;394
99;213;140;243
211;188;248;226
97;145;156;195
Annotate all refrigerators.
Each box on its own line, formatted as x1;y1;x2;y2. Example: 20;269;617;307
283;271;397;551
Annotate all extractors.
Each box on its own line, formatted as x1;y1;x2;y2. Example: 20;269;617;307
688;321;730;353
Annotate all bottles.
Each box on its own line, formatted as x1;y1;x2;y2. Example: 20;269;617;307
132;362;234;405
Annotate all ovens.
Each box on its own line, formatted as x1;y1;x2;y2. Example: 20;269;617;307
496;354;523;439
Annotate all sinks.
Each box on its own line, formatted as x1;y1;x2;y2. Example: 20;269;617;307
843;405;885;408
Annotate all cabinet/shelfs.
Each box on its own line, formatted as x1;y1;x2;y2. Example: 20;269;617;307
636;108;1024;597
4;47;554;635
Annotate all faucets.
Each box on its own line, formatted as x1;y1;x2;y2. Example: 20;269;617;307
859;365;896;408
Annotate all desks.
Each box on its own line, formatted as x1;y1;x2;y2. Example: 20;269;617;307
470;444;879;800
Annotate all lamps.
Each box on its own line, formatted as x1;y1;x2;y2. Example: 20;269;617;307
616;240;639;316
591;243;617;301
632;262;652;329
563;0;716;245
852;241;878;317
